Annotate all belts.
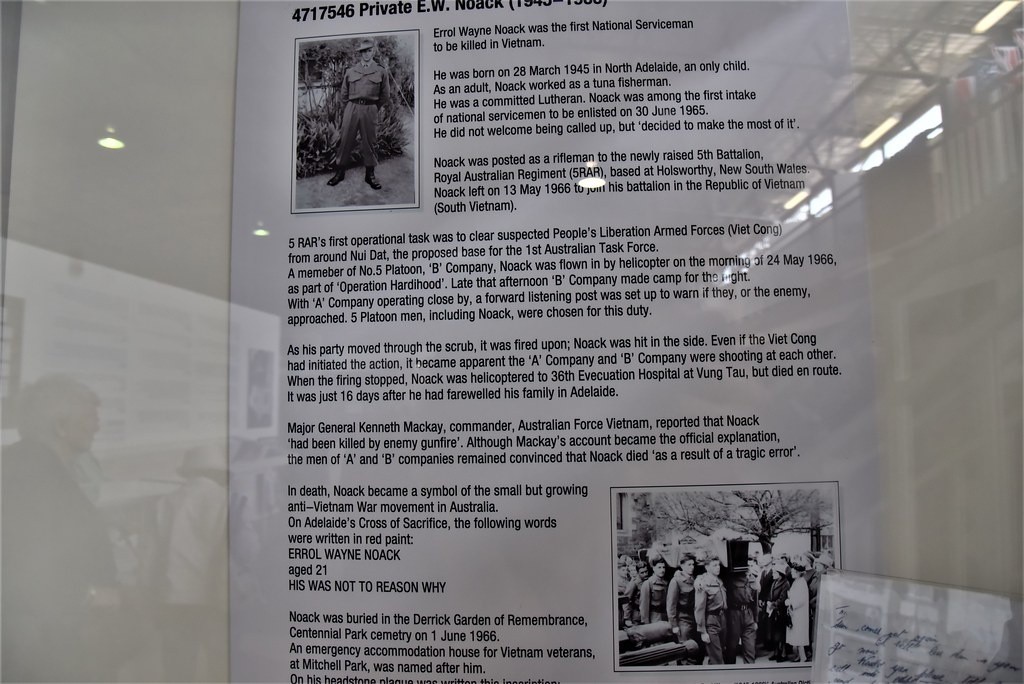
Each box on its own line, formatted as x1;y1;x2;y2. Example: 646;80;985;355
351;99;375;105
730;604;751;611
709;609;724;616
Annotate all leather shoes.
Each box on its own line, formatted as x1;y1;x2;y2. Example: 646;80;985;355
365;173;381;189
327;170;345;185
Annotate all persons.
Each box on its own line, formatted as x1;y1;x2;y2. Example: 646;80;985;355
327;39;390;190
617;548;835;666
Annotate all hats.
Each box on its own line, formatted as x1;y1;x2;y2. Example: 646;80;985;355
773;560;788;575
355;40;374;52
814;555;834;569
